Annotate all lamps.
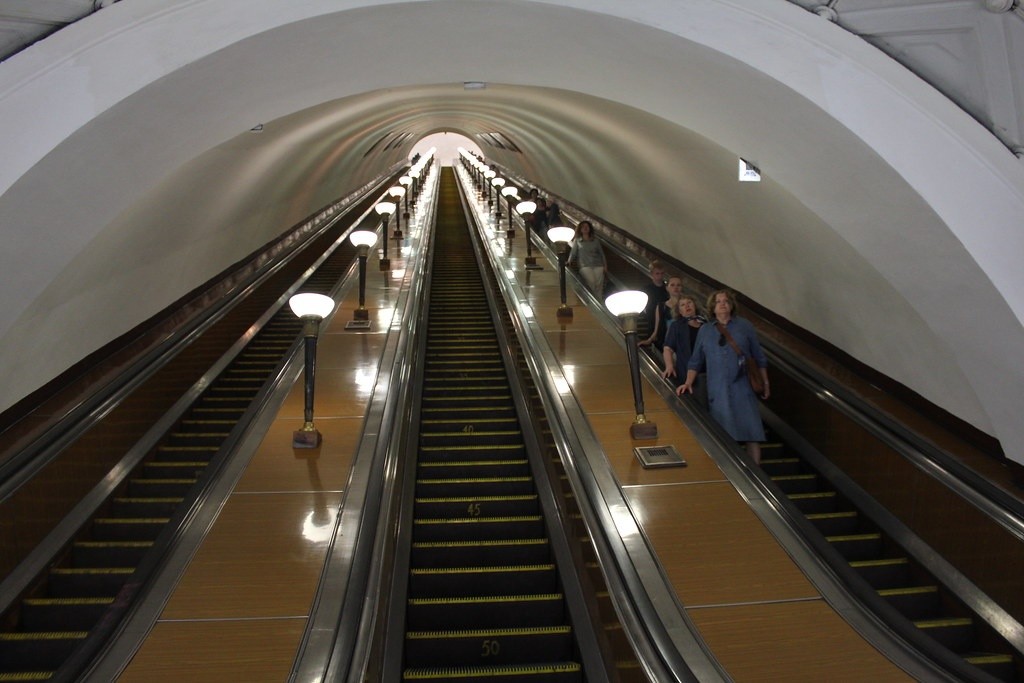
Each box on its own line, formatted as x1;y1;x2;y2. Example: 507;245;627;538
289;292;335;449
457;147;489;198
491;178;505;216
349;230;378;320
408;147;436;205
484;170;496;205
605;289;657;440
516;201;543;270
501;187;517;235
375;202;396;271
399;176;413;218
546;227;575;316
388;186;405;235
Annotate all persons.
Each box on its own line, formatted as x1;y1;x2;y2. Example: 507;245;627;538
662;295;708;383
412;152;421;165
534;198;553;242
635;274;683;345
675;290;770;468
528;188;542;207
549;203;564;229
643;260;668;342
564;221;607;299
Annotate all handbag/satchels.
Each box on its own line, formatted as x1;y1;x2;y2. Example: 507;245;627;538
748;359;764;391
